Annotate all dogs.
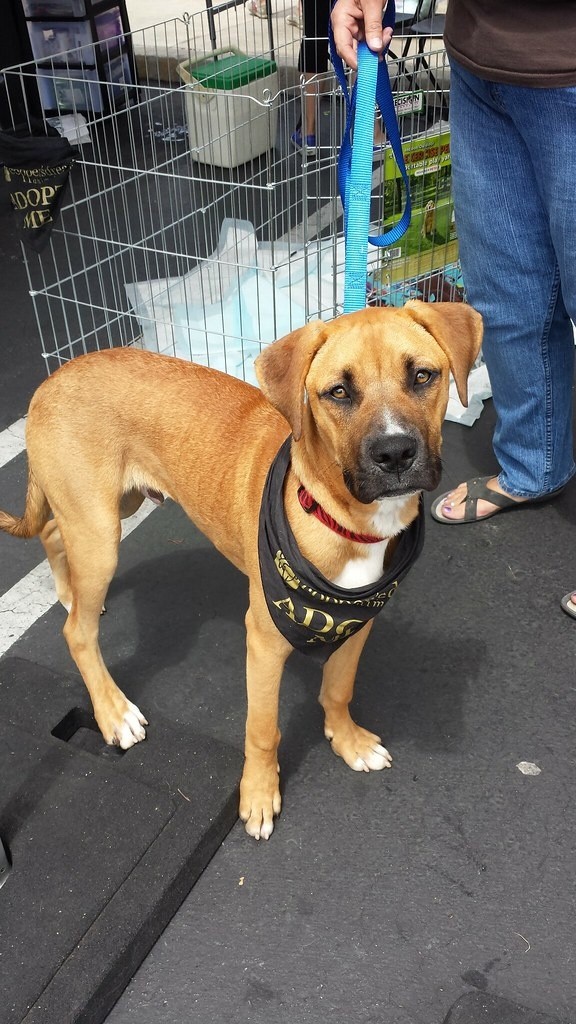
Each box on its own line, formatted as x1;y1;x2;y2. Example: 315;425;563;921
421;200;436;239
0;299;484;841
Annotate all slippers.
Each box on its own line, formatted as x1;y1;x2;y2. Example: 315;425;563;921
430;474;566;524
561;591;576;618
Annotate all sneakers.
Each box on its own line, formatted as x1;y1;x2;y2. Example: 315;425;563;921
374;133;411;151
291;129;318;156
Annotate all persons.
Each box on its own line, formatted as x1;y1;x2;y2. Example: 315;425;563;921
246;0;304;30
331;0;576;625
291;0;396;157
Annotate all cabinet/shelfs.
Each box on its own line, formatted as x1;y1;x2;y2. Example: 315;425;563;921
8;0;142;122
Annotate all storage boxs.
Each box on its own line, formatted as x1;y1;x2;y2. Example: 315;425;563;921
177;44;281;169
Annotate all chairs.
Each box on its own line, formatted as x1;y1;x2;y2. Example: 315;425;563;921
387;0;448;125
